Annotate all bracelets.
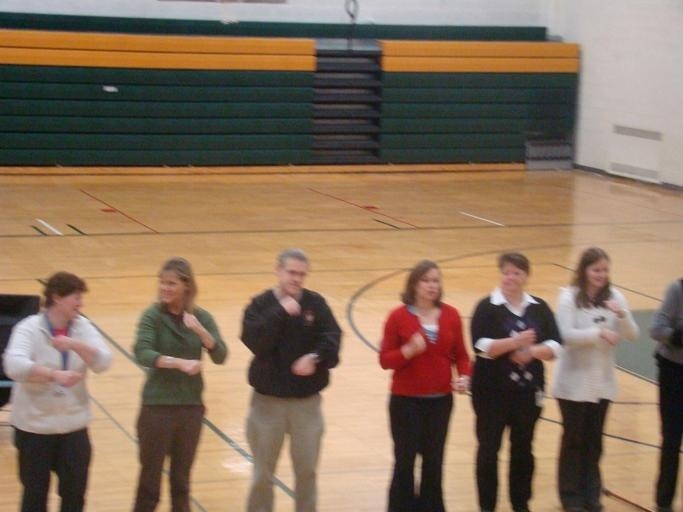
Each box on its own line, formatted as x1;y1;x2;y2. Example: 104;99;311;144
308;352;321;365
46;368;58;383
163;354;173;367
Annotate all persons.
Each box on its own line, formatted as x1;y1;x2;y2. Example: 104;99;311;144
0;270;112;511
471;251;561;511
648;278;683;512
240;249;343;511
377;260;471;511
132;255;228;511
546;248;640;511
